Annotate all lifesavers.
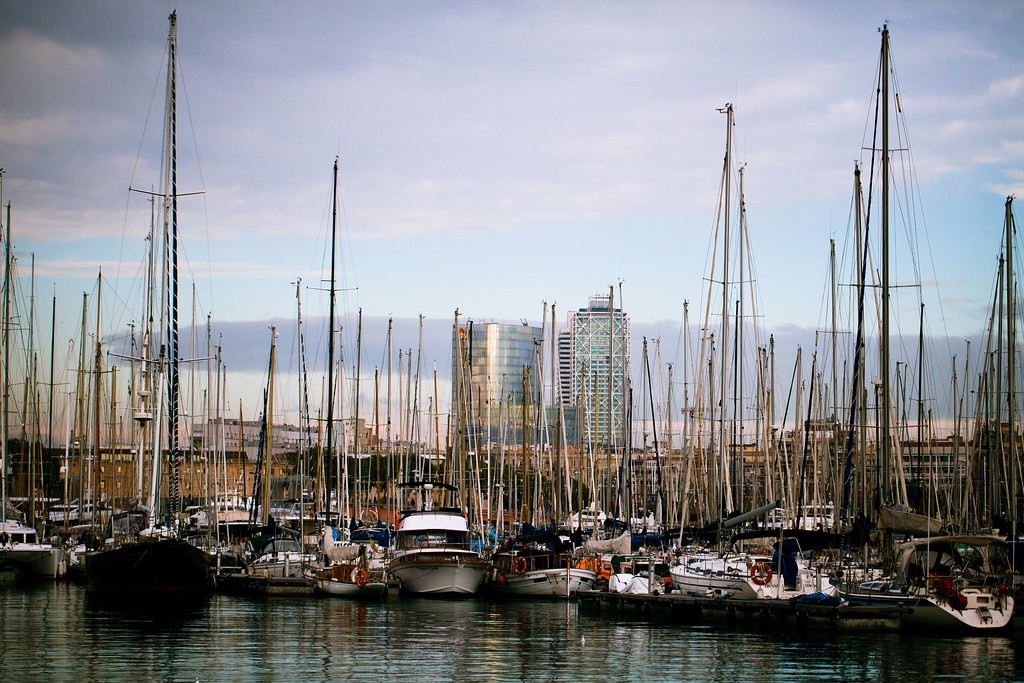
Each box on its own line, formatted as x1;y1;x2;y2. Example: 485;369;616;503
750;563;773;585
514;556;527;575
355;568;368;588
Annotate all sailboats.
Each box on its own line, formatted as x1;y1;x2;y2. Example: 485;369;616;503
84;8;214;617
0;7;1024;635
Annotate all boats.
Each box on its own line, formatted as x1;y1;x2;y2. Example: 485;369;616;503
387;469;493;601
